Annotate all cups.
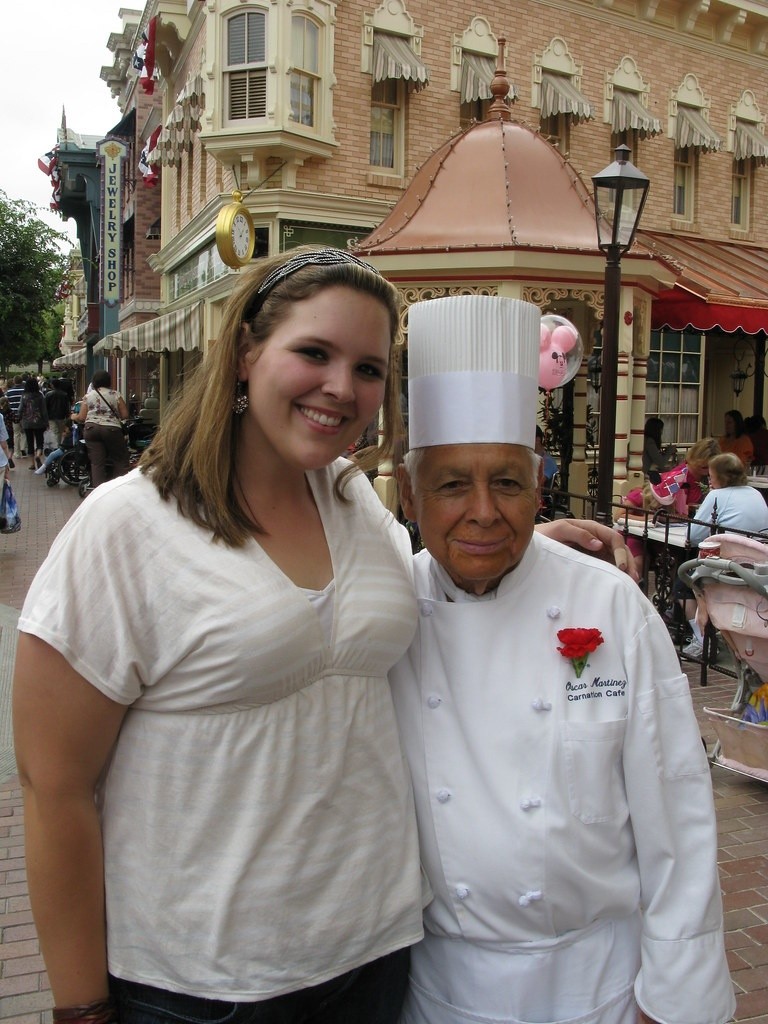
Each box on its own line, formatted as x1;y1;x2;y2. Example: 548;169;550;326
698;540;721;559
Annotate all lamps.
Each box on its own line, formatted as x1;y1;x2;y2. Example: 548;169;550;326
145;228;161;240
728;336;758;397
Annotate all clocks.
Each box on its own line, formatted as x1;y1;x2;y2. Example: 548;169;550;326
214;189;255;269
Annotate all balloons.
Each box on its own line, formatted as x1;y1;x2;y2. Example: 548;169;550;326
538;314;584;391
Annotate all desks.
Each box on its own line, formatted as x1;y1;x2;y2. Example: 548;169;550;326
616;517;691;644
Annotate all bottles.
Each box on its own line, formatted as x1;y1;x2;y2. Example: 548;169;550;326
753;467;756;477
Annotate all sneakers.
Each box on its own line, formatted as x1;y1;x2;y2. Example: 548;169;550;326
679;639;719;662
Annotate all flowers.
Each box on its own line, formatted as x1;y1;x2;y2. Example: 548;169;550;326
554;625;606;678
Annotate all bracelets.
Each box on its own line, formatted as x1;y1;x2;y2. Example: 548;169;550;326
52;998;113;1024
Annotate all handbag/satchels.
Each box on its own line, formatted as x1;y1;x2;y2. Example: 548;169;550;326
0;477;22;534
121;422;128;436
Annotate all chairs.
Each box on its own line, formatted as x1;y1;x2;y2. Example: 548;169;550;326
743;426;768;476
141;398;160;425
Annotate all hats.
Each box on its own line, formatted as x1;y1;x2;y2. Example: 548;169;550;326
650;463;688;505
407;297;541;448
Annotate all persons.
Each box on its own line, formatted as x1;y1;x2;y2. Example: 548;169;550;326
0;371;75;497
613;467;688;577
534;424;558;494
677;452;768;660
11;243;639;1024
642;417;675;473
70;370;130;487
672;437;723;515
718;410;768;465
387;295;738;1024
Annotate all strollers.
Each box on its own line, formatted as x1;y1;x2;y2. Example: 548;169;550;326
676;533;768;783
44;415;91;497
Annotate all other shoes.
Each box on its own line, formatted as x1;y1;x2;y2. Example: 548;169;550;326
35;464;47;475
21;450;27;456
8;460;15;468
29;465;35;470
36;456;43;469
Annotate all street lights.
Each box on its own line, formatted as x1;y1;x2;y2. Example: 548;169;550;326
591;143;650;530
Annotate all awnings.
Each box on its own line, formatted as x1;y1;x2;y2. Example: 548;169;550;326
675;105;723;155
539;71;596;125
93;299;204;359
611;88;663;141
461;51;520;107
371;32;429;93
733;120;768;167
622;227;768;334
52;347;86;369
146;73;205;169
